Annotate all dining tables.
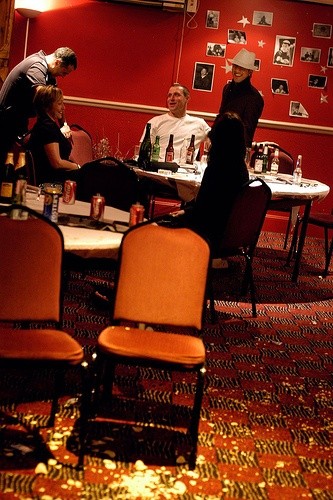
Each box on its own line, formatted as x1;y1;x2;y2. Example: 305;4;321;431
15;195;148;260
126;162;330;283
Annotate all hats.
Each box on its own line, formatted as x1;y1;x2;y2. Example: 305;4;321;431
228;48;257;70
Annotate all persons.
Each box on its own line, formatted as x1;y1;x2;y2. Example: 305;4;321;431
28;84;92;192
0;44;77;180
196;47;265;172
91;109;249;308
190;10;333;125
139;82;213;200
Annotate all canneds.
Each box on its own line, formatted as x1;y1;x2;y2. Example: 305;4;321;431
129;202;145;227
43;188;58;225
89;196;105;221
63;180;76;205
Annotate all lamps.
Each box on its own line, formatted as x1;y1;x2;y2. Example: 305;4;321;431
16;8;42;61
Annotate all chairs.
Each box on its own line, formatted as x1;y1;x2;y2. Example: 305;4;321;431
0;203;85;472
76;214;212;470
12;124;333;322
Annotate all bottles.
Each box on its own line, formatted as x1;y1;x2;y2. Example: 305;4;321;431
244;142;279;174
0;153;16;204
186;134;196;163
137;122;151;169
13;152;28;206
165;134;174;163
292;155;302;183
151;135;160;161
179;138;188;163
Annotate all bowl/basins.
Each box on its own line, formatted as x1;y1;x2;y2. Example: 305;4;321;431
24;185;41;201
40;183;62;195
